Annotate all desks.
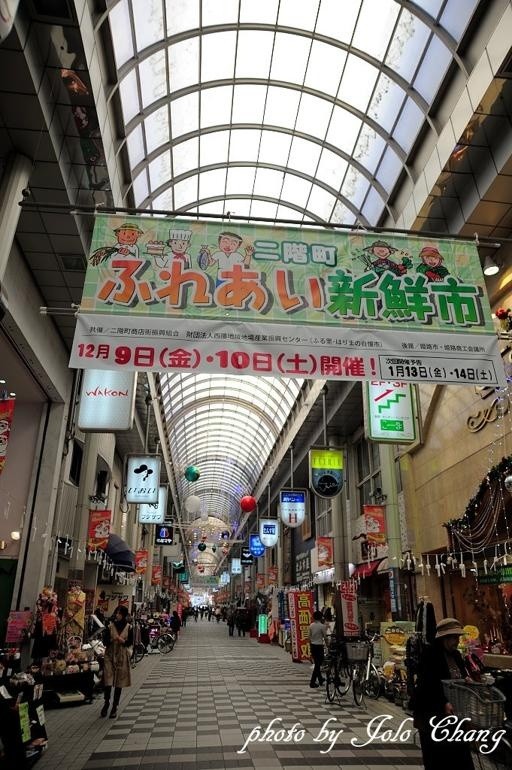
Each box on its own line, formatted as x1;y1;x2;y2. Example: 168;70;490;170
36;670;94;709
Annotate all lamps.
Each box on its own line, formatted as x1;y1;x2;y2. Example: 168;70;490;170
11;531;20;540
483;255;499;276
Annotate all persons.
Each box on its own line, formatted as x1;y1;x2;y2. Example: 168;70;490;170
60;585;87;647
415;595;437;646
410;618;491;770
170;604;257;641
307;611;330;688
31;583;60;663
100;604;134;718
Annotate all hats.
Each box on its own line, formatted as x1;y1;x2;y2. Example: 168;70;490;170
435;618;466;639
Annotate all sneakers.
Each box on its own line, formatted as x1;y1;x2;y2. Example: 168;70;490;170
310;678;325;688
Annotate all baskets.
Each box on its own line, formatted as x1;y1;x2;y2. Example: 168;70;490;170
442;680;506;729
345;642;368;660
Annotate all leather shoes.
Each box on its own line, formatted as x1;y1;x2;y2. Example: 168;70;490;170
101;702;117;718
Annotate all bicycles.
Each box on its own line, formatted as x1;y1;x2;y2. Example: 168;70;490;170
154;618;176;654
439;668;511;770
322;632;353;706
129;638;147;666
345;620;385;710
